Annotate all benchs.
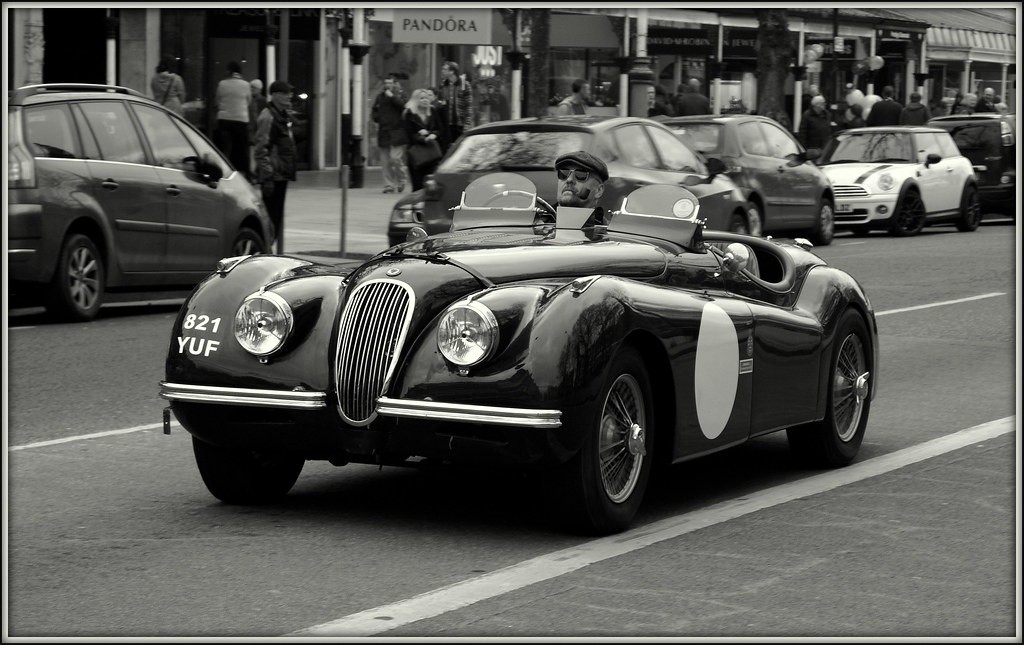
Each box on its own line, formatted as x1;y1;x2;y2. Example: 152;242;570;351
698;236;760;276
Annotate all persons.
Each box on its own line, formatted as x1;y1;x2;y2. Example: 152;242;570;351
253;81;298;254
439;62;474;143
248;79;266;181
900;93;930;125
648;78;710;117
371;79;450;194
150;54;185;115
798;84;867;149
558;78;592;116
554;150;608;225
215;61;249;171
868;87;902;125
928;87;1001;116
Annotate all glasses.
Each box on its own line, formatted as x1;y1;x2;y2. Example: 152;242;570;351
557;168;601;185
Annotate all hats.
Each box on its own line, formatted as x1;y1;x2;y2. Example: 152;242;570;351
269;80;296;93
554;151;610;182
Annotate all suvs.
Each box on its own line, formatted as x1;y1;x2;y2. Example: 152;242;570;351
7;82;279;324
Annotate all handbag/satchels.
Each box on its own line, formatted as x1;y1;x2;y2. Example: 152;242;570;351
407;139;441;167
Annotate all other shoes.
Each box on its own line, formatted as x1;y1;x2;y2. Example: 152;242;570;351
384;188;395;193
398;184;404;192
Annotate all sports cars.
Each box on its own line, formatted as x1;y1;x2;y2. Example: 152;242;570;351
155;171;879;536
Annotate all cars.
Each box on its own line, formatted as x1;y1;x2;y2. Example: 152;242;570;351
808;124;983;230
386;113;753;252
922;111;1016;224
652;113;836;249
